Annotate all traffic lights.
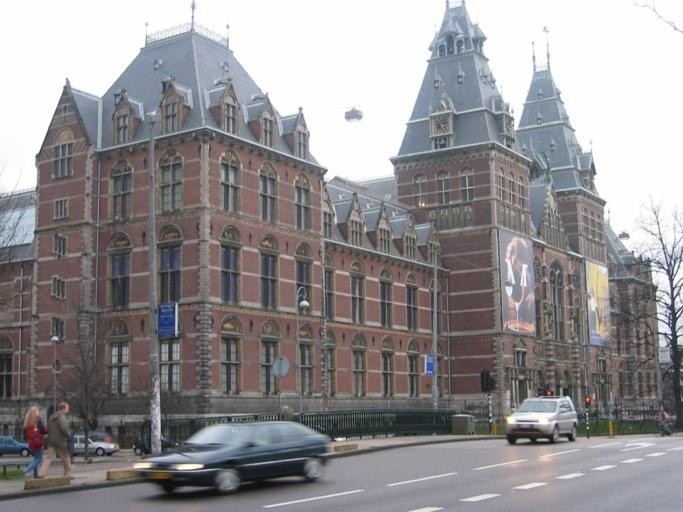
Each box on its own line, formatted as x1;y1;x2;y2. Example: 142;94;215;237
584;396;591;409
547;391;553;398
537;385;546;396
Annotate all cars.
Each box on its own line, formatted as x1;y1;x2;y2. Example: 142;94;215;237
0;435;33;458
68;433;122;456
131;433;183;456
132;420;334;499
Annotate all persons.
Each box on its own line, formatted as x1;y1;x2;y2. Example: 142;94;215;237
18;406;49;479
656;410;673;436
35;401;74;479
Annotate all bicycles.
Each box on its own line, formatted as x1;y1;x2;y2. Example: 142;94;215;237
653;419;672;436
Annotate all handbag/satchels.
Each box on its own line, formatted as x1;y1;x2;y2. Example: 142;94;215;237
32;431;42;449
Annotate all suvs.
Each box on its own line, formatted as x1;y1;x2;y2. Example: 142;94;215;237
502;394;579;446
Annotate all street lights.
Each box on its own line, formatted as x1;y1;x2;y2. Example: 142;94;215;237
294;283;310;413
50;337;61;415
